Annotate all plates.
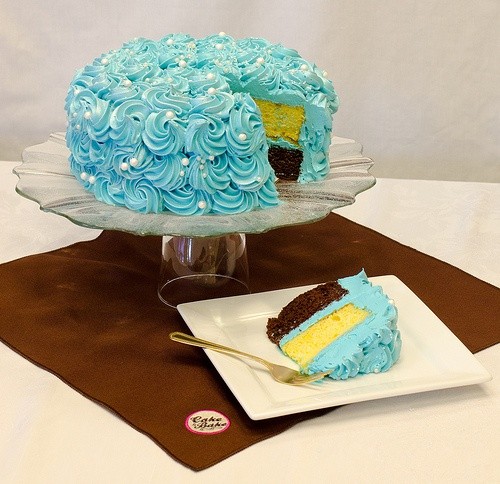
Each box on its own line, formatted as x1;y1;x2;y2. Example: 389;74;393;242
177;274;489;421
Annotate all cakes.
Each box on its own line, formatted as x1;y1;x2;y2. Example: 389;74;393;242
64;34;340;216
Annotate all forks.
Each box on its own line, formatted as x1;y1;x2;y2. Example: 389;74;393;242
169;330;332;387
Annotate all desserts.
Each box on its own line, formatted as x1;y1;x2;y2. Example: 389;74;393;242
266;267;402;379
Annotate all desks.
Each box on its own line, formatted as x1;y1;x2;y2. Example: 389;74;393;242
0;160;500;484
